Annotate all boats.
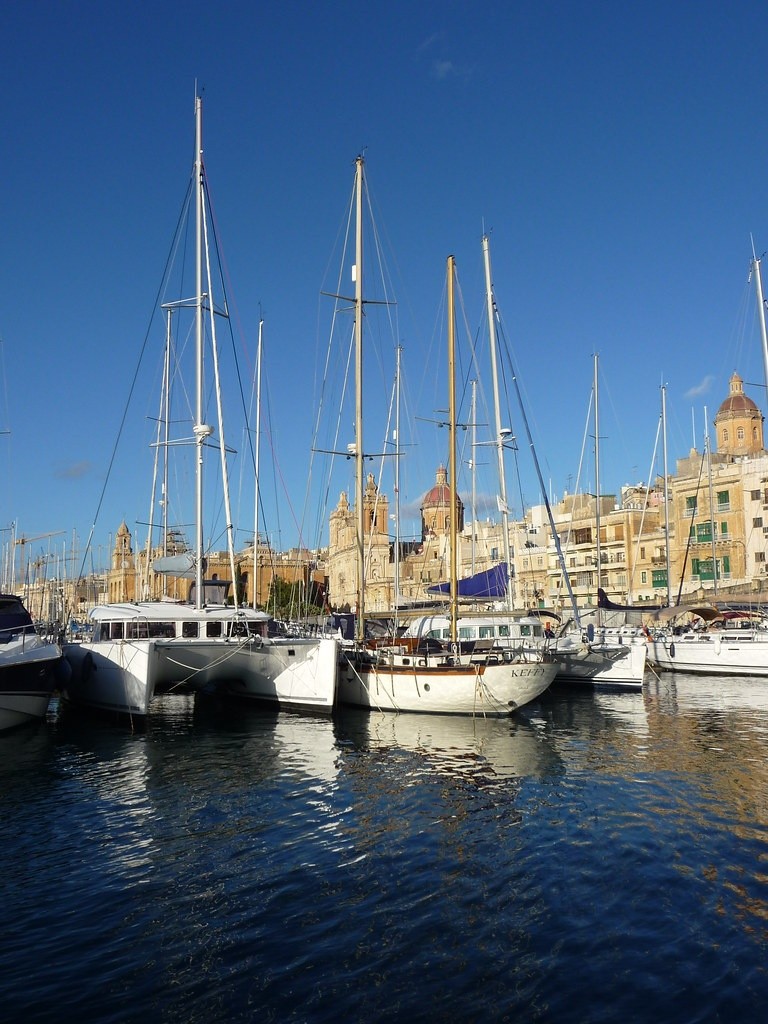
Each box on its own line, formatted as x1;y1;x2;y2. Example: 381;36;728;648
0;592;74;720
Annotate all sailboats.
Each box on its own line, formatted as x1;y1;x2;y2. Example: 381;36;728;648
50;91;768;723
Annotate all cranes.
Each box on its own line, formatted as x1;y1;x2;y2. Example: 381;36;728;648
13;529;68;587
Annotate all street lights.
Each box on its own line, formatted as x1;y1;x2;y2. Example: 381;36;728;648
732;541;753;641
